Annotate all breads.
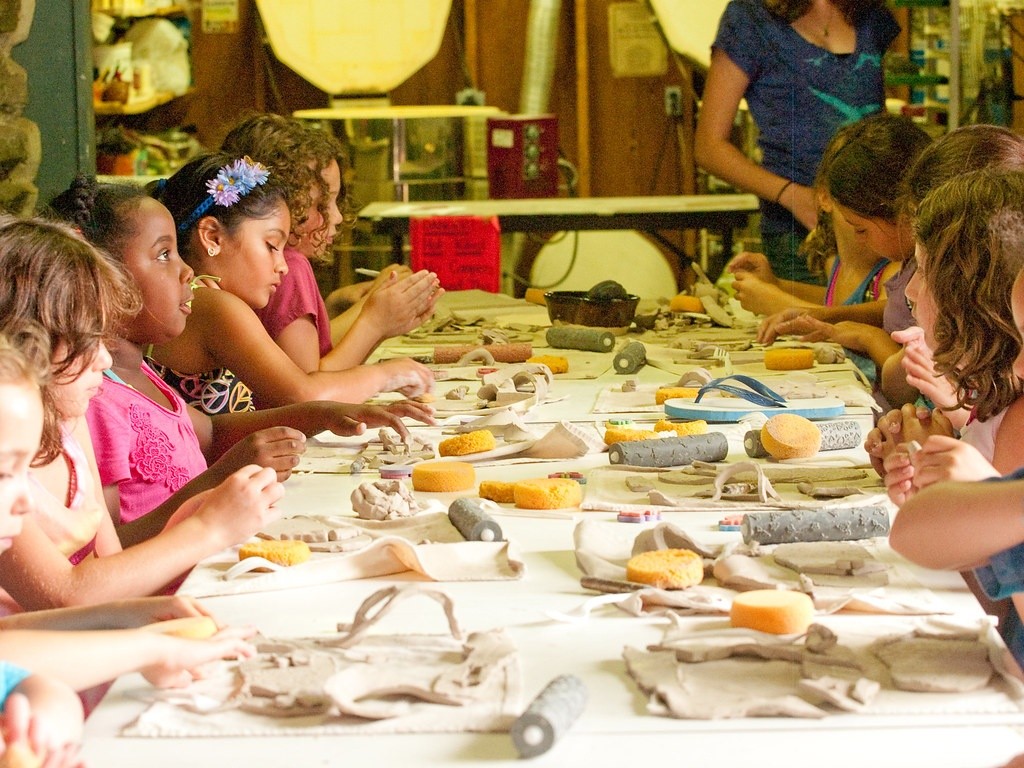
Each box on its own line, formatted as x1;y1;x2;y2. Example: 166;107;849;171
759;414;821;459
604;420;707;447
655;387;699;405
479;477;582;509
670;295;704;312
730;588;815;634
411;462;475;492
764;349;815;370
625;548;704;589
239;539;310;572
439;429;496;456
525;355;569;374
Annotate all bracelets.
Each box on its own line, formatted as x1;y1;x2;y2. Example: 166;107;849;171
938;388;970;412
775;180;794;204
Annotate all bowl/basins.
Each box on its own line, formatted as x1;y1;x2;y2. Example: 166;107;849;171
543;290;640;327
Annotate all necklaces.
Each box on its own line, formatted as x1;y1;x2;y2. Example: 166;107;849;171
806;6;835;38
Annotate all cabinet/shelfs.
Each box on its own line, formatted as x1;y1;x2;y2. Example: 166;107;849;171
290;104;506;270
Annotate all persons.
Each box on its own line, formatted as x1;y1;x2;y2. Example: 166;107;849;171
0;113;444;766
723;112;1024;676
692;0;904;288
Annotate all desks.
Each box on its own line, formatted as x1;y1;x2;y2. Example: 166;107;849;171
70;314;1024;767
354;193;761;315
